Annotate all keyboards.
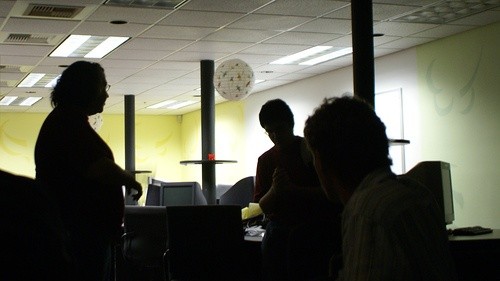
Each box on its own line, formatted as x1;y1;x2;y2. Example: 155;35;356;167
453;226;493;236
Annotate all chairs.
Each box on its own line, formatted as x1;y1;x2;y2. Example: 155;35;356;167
119;205;262;281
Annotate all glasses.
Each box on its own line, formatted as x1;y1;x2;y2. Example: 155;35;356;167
105;84;110;92
265;131;270;136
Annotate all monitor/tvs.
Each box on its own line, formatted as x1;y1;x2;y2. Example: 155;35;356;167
395;162;453;224
219;175;256;204
146;181;207;207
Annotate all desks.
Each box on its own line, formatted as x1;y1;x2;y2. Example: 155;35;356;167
448;229;500;281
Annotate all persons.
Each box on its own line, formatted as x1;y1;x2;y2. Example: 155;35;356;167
253;98;326;281
304;92;456;281
35;61;143;281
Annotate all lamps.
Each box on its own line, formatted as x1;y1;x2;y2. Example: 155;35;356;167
213;58;256;102
